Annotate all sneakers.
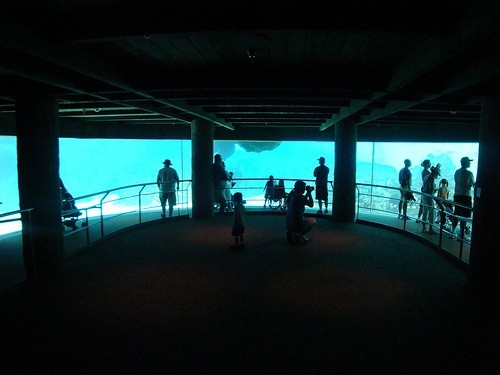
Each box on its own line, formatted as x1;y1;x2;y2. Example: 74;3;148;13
161;213;166;218
300;235;312;242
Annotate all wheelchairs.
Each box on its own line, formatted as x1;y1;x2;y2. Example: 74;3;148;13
268;187;291;210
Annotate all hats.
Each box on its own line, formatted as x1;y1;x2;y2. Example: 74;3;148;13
460;157;474;161
431;168;441;176
317;157;325;160
162;160;173;165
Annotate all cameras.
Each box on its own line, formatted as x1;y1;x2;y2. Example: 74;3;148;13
306;186;314;191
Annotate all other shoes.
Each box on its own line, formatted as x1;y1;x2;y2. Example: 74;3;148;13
398;214;409;220
428;229;438;235
422;228;427;233
416;220;422;223
317;210;322;213
447;233;454;238
325;210;328;213
465;227;470;234
435;221;439;224
457;236;466;242
230;244;246;248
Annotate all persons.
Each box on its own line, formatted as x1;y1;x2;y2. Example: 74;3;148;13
213;154;236;215
286;181;316;243
438;179;453;223
274;180;288;209
313;157;330;214
447;157;475;242
59;177;69;231
263;175;274;208
232;192;246;250
415;159;436;223
421;166;441;234
397;159;412;220
157;159;179;222
